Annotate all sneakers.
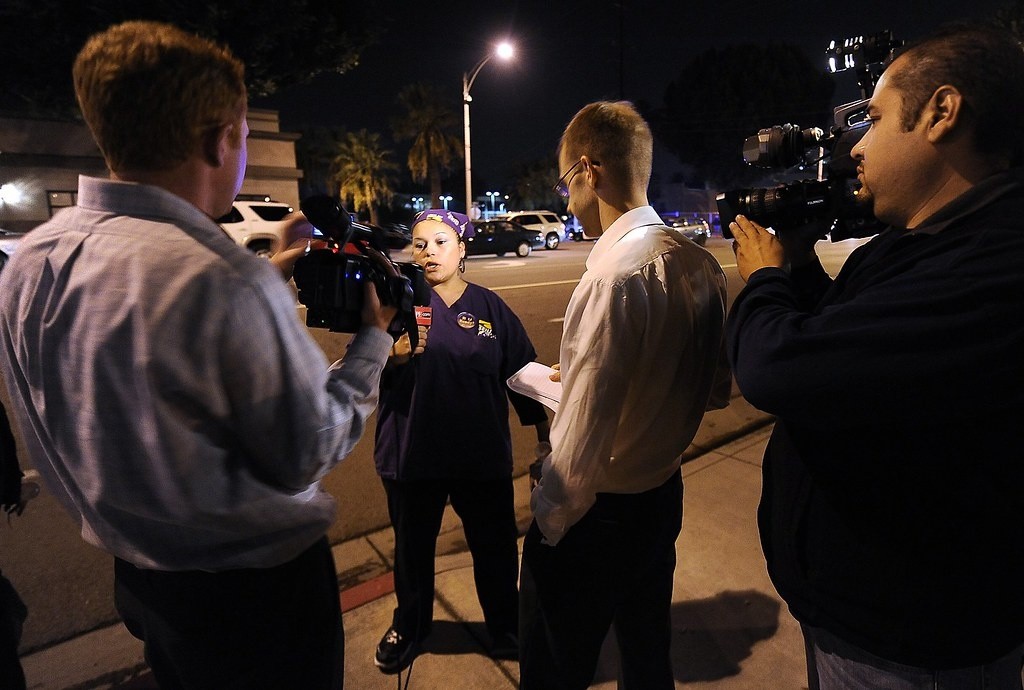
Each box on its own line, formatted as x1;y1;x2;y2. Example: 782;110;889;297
374;625;413;669
491;630;519;655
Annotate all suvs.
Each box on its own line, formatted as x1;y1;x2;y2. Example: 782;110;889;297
492;209;565;250
217;201;294;257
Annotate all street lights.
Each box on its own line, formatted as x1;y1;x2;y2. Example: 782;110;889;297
411;197;423;214
486;191;500;211
439;196;453;210
462;42;512;222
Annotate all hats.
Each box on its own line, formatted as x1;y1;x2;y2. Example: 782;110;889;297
410;208;476;241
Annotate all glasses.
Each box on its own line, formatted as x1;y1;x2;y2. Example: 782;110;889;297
551;158;601;198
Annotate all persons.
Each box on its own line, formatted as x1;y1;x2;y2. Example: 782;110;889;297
0;22;400;690
519;103;733;690
726;32;1024;690
372;209;553;670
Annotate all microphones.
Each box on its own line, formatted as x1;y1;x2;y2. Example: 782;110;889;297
744;123;821;170
351;221;407;250
415;282;434;329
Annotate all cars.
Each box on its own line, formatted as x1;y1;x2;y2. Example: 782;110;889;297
464;221;545;258
0;228;27;273
663;216;711;248
564;218;583;240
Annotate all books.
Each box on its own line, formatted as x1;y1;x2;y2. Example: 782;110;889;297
506;362;563;414
